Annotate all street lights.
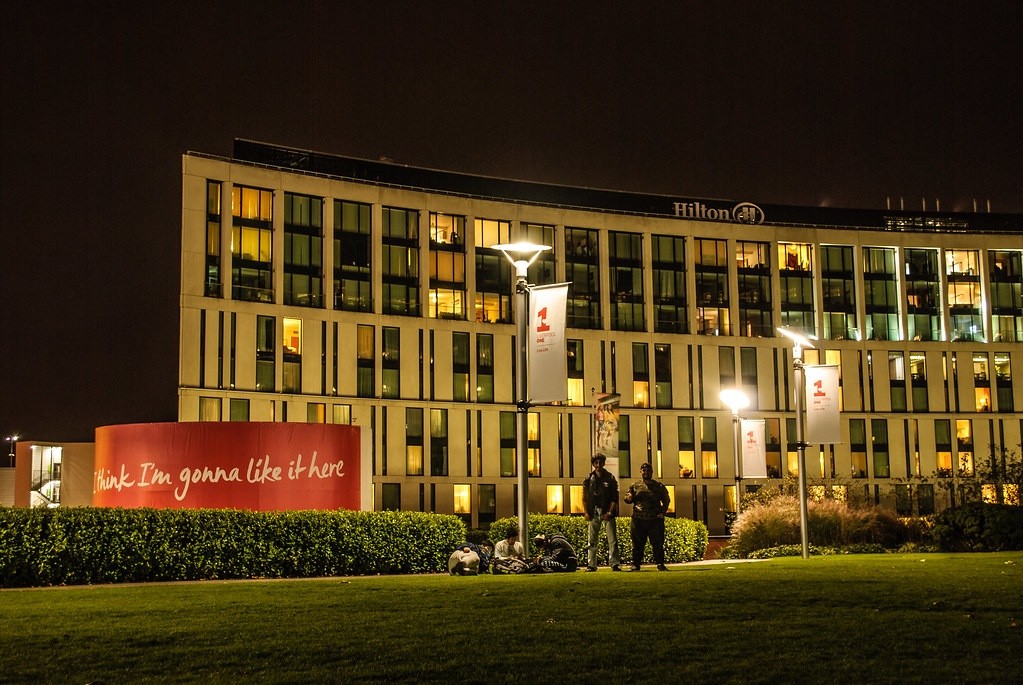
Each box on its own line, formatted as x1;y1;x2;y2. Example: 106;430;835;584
777;325;817;558
492;239;552;556
5;436;19;467
719;388;750;514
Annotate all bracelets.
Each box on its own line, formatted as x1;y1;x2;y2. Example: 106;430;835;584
607;512;613;515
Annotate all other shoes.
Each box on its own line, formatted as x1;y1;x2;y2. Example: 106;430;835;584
613;566;620;571
658;565;669;571
630;565;640;570
586;567;595;572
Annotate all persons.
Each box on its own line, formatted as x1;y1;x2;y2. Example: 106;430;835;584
493;527;537;574
582;452;622;572
623;463;670;571
533;534;577;572
447;540;496;576
